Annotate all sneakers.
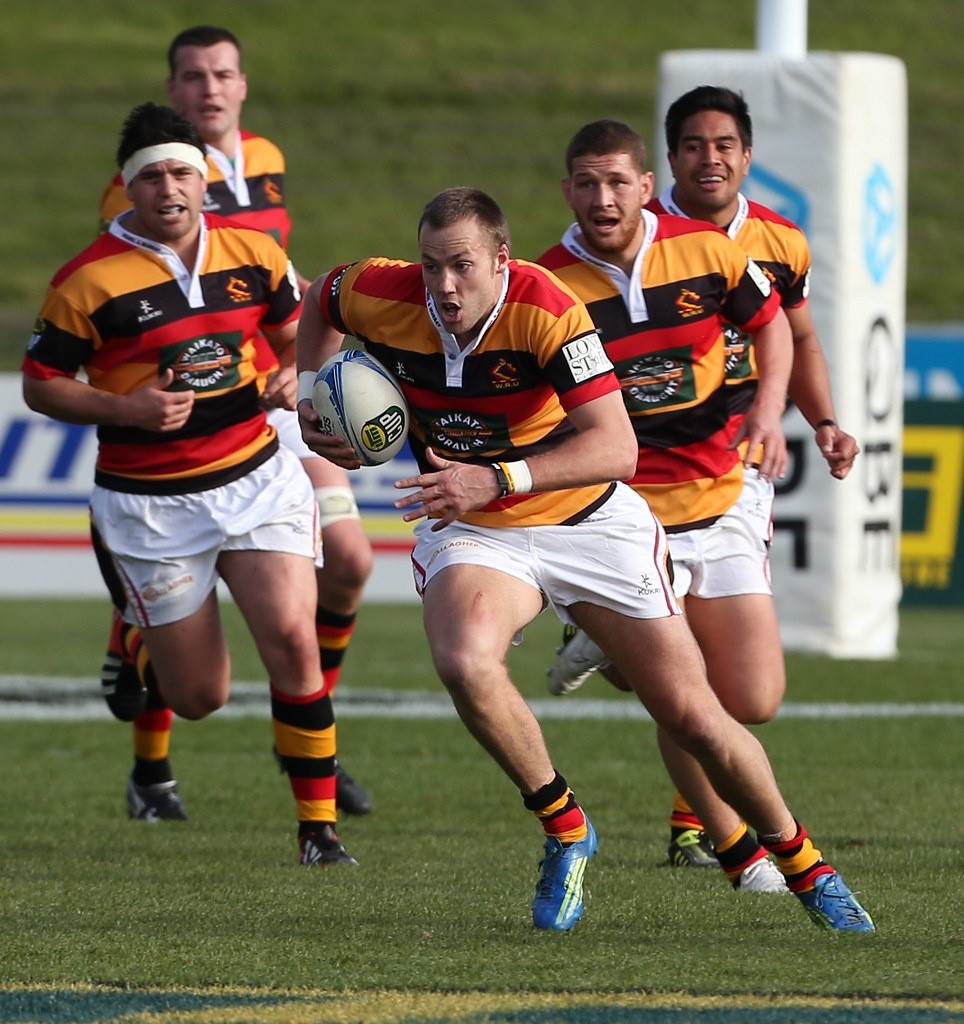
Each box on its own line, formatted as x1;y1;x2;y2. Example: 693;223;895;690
532;812;596;933
544;619;614;697
101;639;147;721
296;826;361;868
666;822;720;869
126;771;188;823
795;870;873;933
272;741;370;817
730;858;792;895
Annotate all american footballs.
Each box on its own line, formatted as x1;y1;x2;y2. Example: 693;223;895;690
312;348;412;467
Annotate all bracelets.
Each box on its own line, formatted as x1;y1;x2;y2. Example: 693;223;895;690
814;418;837;432
488;459;535;499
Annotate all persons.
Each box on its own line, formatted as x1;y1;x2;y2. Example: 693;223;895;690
94;25;378;821
294;185;877;942
529;82;860;898
13;94;365;875
